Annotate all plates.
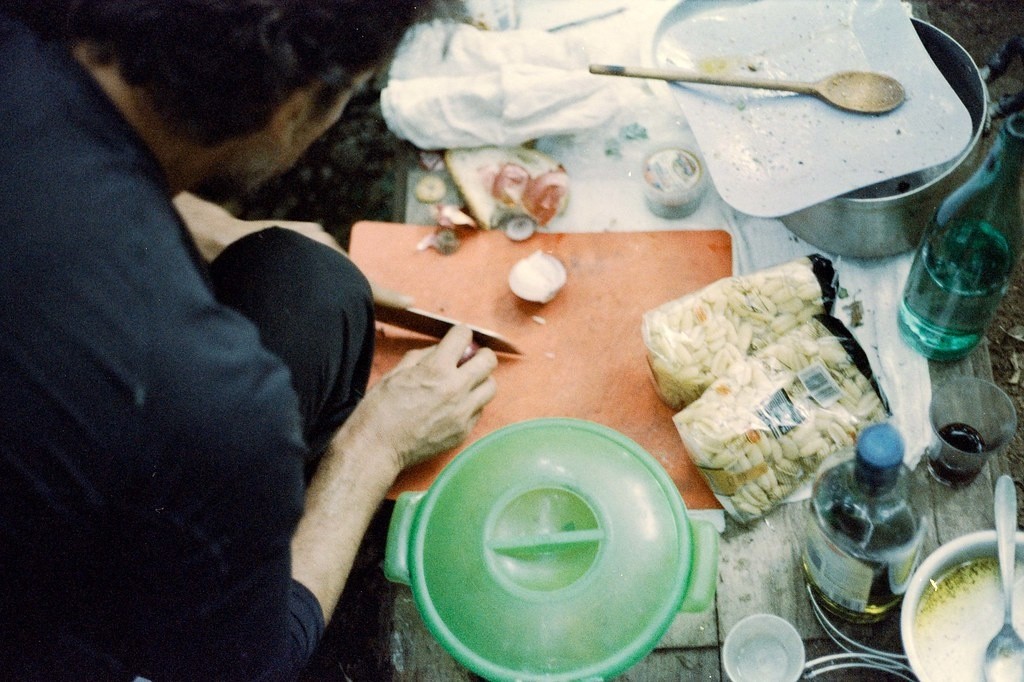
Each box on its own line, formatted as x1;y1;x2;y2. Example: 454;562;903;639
652;0;972;217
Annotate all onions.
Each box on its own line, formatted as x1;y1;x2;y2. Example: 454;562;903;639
509;250;567;304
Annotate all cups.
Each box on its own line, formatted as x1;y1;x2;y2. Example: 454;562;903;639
724;614;806;682
642;146;707;219
925;375;1017;488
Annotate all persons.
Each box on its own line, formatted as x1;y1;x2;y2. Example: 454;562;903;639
0;1;502;682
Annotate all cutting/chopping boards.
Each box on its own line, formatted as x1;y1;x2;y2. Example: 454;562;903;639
349;220;732;509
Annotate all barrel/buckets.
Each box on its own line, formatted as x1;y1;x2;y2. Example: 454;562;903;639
781;16;991;256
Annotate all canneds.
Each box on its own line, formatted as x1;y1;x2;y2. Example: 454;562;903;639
638;140;705;219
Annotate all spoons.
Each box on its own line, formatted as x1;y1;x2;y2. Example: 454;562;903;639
981;474;1024;682
589;63;906;113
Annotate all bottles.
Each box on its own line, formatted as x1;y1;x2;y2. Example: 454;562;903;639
802;424;917;624
899;113;1024;363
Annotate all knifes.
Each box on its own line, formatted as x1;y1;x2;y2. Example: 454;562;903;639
374;300;521;357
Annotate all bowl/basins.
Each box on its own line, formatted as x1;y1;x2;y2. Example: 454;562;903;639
383;418;718;682
900;530;1024;682
